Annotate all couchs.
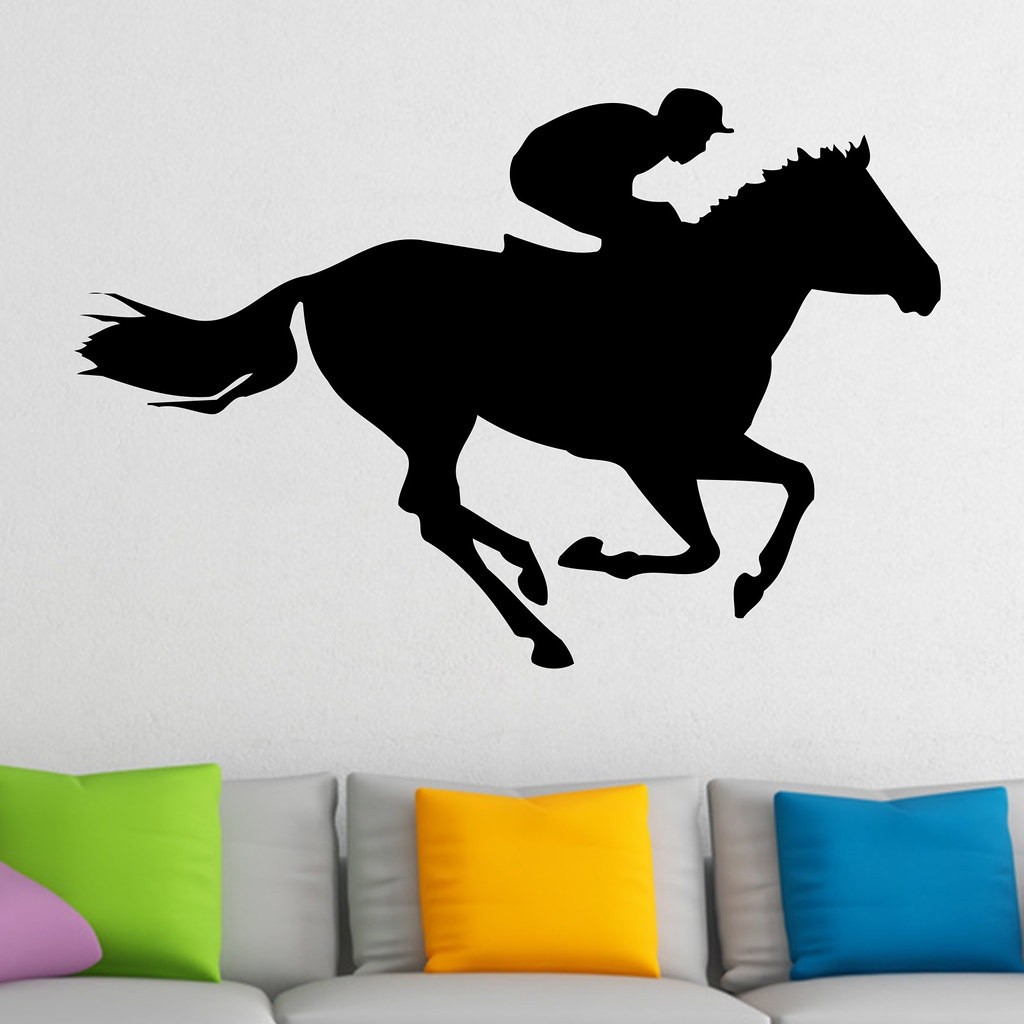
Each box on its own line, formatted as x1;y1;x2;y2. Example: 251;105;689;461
0;760;1024;1024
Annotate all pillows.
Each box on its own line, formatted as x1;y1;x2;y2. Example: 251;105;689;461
220;772;341;1002
415;785;661;979
704;776;1024;992
347;772;709;982
0;865;102;982
0;766;223;984
775;787;1024;980
